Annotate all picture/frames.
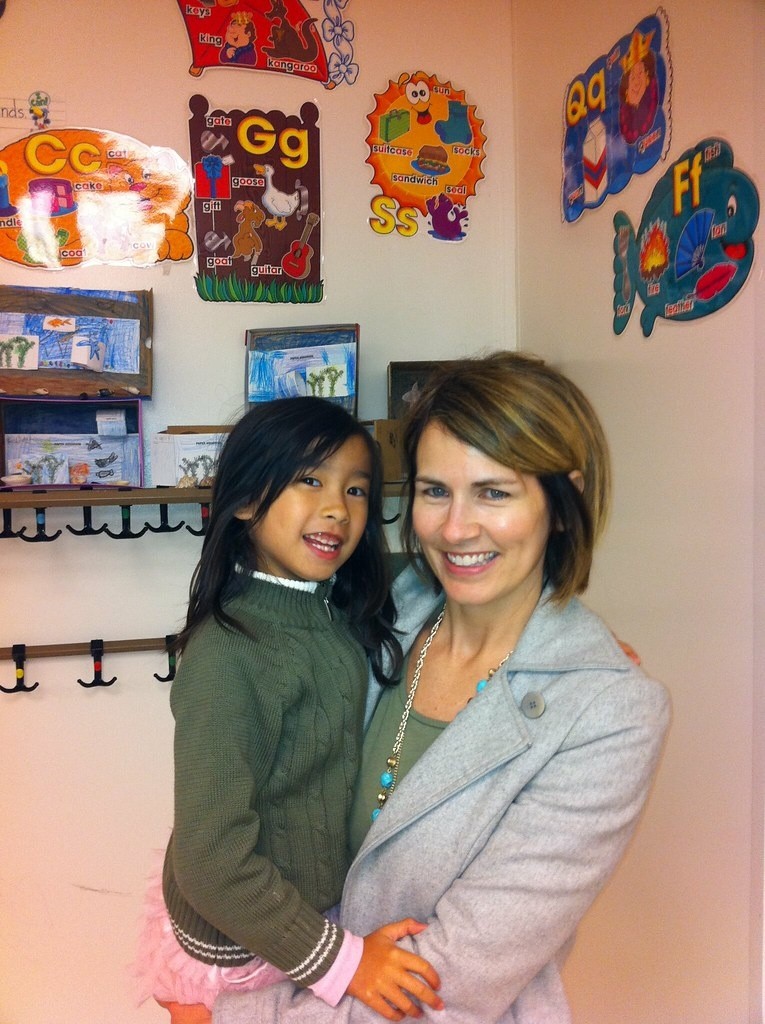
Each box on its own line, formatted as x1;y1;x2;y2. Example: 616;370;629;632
386;361;446;419
243;324;360;417
0;284;154;401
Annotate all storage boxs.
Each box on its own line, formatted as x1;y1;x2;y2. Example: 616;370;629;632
0;397;142;492
360;418;413;498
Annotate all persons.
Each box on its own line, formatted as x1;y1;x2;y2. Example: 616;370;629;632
155;350;668;1023
128;394;643;1022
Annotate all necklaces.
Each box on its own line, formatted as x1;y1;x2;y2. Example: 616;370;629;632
371;602;515;823
